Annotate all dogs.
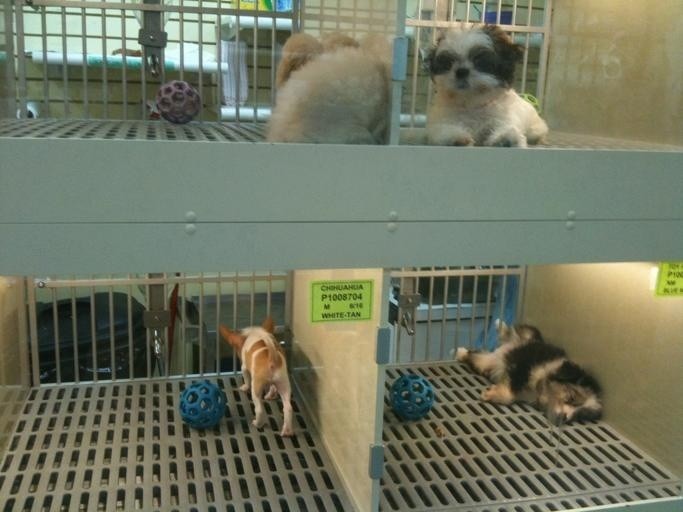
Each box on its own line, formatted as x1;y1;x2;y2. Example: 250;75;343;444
217;316;295;440
419;21;550;148
262;29;390;145
447;316;605;427
